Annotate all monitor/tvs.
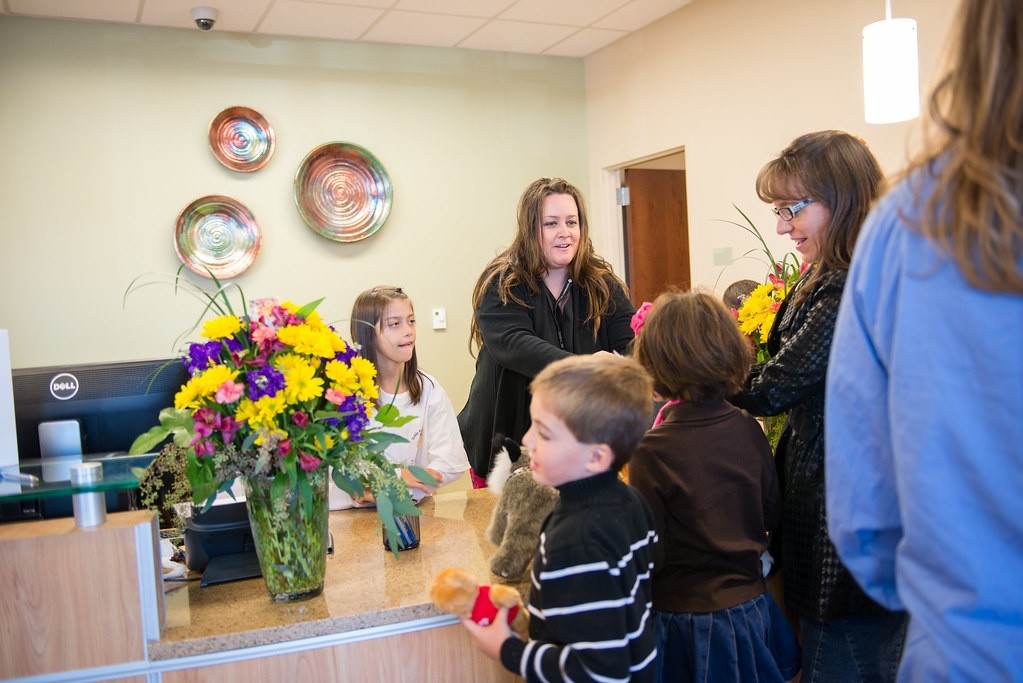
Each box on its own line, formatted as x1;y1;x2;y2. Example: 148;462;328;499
11;359;192;530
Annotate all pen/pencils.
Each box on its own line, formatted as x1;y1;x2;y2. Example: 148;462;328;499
2;471;41;483
386;514;416;549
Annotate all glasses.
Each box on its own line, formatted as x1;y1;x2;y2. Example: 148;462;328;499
773;195;818;221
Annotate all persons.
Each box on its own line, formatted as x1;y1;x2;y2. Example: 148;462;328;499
458;0;1023;683
328;286;471;512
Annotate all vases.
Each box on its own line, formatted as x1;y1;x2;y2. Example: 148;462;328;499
243;463;330;604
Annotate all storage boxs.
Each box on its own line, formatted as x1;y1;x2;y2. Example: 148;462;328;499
183;496;255;573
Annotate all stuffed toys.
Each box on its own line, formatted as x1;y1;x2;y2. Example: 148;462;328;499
430;566;530;641
486;438;559;581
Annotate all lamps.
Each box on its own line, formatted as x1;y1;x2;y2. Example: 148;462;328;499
863;0;919;125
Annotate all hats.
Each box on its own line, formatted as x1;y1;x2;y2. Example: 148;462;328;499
722;280;761;309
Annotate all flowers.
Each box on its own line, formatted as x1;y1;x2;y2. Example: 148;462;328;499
120;259;442;561
629;301;653;335
712;200;808;455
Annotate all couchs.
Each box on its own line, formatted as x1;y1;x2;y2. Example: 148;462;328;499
16;392;176;515
